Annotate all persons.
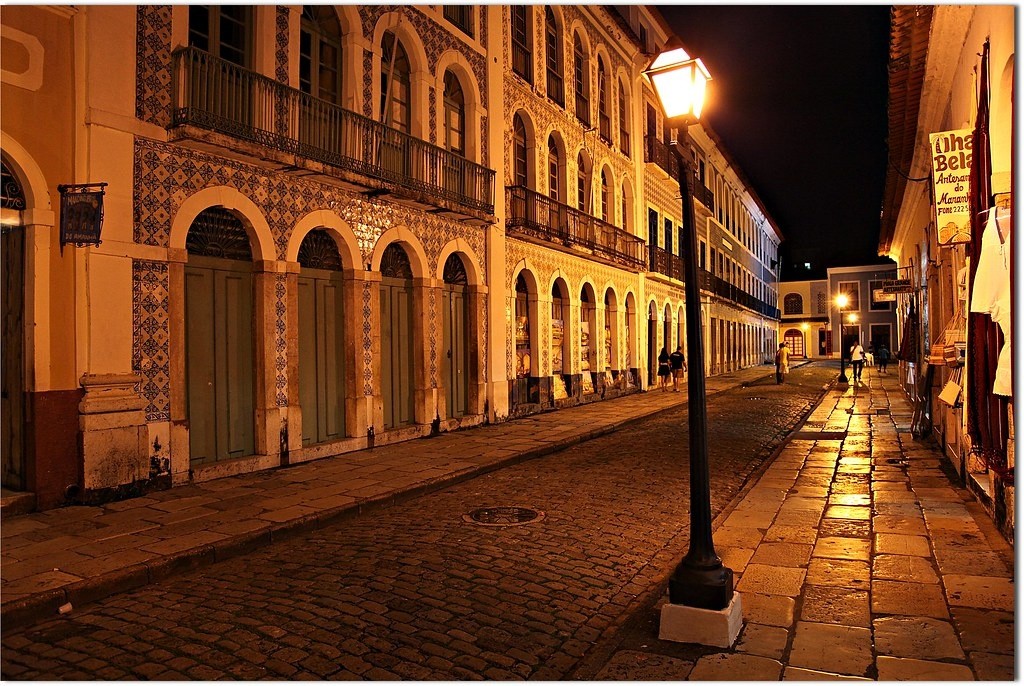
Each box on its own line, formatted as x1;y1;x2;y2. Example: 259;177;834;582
877;344;889;374
657;346;686;394
775;341;790;385
849;339;865;380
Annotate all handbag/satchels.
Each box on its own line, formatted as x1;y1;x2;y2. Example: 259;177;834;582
938;368;964;408
929;305;966;366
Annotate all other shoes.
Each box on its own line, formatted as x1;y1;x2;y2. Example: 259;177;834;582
673;389;680;393
663;389;668;392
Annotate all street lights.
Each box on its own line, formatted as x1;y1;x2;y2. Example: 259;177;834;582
835;294;849;382
639;35;733;611
